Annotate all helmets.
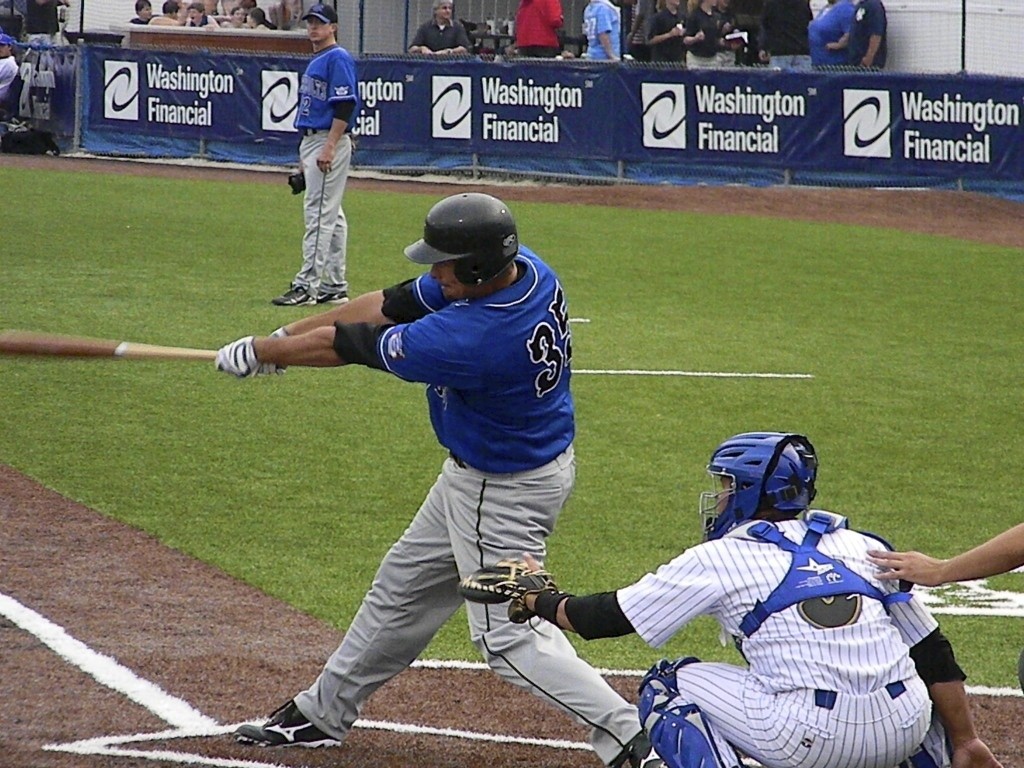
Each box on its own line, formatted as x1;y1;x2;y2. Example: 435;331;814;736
403;192;520;286
697;430;820;542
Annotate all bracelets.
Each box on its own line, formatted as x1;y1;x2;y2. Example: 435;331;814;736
532;589;569;629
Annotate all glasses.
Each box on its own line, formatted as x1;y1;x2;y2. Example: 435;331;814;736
440;5;453;10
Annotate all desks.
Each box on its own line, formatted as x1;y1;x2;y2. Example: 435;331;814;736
566;35;589;59
470;33;521;61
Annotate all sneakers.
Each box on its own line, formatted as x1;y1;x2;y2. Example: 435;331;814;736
271;281;317;308
605;729;668;768
316;290;349;305
233;698;342;750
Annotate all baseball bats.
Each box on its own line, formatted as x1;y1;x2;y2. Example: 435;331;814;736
0;331;288;374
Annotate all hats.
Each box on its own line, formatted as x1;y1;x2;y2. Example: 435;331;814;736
301;3;337;23
0;34;13;45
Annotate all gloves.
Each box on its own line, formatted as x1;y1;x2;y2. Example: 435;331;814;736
212;334;260;379
258;327;293;376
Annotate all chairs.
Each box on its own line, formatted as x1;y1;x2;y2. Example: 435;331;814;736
0;75;21;123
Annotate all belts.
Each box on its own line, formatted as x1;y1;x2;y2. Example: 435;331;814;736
815;676;907;711
302;128;331;136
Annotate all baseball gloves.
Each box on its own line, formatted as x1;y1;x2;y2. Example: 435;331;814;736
458;559;556;623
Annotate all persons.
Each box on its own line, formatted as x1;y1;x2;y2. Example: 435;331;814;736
867;519;1024;585
516;0;564;59
0;34;20;104
624;0;747;67
497;431;1002;768
409;0;472;55
582;0;622;60
272;5;357;306
222;194;665;767
130;0;276;31
809;0;887;69
